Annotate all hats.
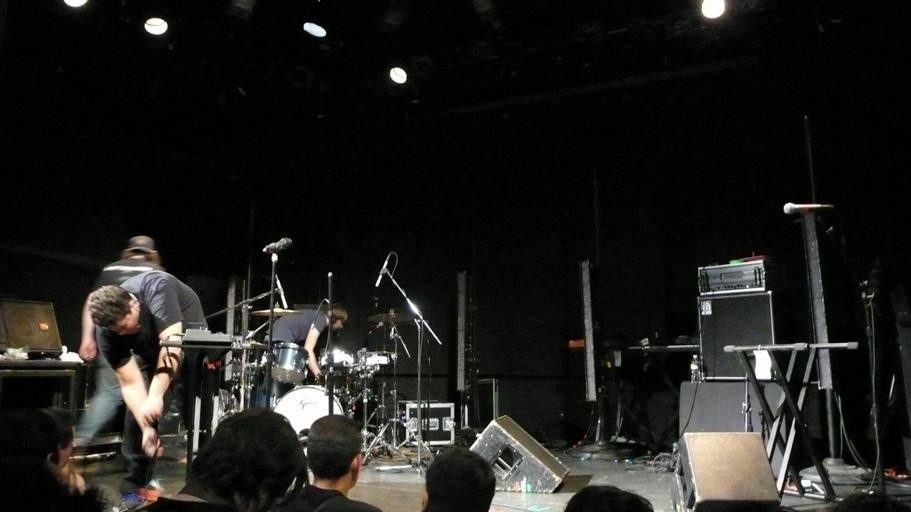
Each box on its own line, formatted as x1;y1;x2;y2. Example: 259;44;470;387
124;236;154;253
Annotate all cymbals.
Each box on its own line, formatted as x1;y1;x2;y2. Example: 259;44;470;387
370;314;416;322
253;309;303;316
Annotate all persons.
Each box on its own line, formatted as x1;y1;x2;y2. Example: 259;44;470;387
134;403;311;512
70;232;170;502
420;445;499;511
87;268;212;502
0;402;108;511
264;414;386;512
254;300;349;412
566;484;654;511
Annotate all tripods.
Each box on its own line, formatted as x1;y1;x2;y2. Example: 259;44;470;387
344;319;437;476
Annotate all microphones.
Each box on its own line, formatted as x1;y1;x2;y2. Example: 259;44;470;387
263;237;292;253
783;202;834;214
375;260;389;288
276;276;289;310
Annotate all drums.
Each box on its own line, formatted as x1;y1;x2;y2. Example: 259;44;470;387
271;341;308;383
274;385;344;456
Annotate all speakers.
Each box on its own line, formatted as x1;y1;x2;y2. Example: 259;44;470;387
470;415;570;494
670;431;782;512
697;293;775;381
0;297;63;359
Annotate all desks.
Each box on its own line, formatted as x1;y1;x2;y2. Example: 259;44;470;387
0;359;81;420
